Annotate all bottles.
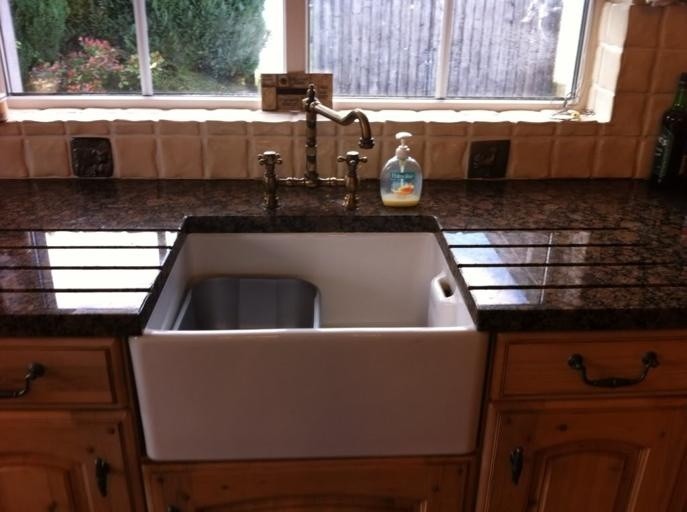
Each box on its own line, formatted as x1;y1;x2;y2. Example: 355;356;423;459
647;72;687;201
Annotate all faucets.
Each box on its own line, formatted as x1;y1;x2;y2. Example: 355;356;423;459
301;82;375;182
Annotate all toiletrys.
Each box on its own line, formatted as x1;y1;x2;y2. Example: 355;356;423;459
378;130;422;206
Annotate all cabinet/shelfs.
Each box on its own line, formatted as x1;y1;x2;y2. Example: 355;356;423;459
0;337;143;512
138;456;478;510
478;332;687;511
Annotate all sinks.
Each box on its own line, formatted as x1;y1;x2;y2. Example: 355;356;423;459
133;213;487;460
